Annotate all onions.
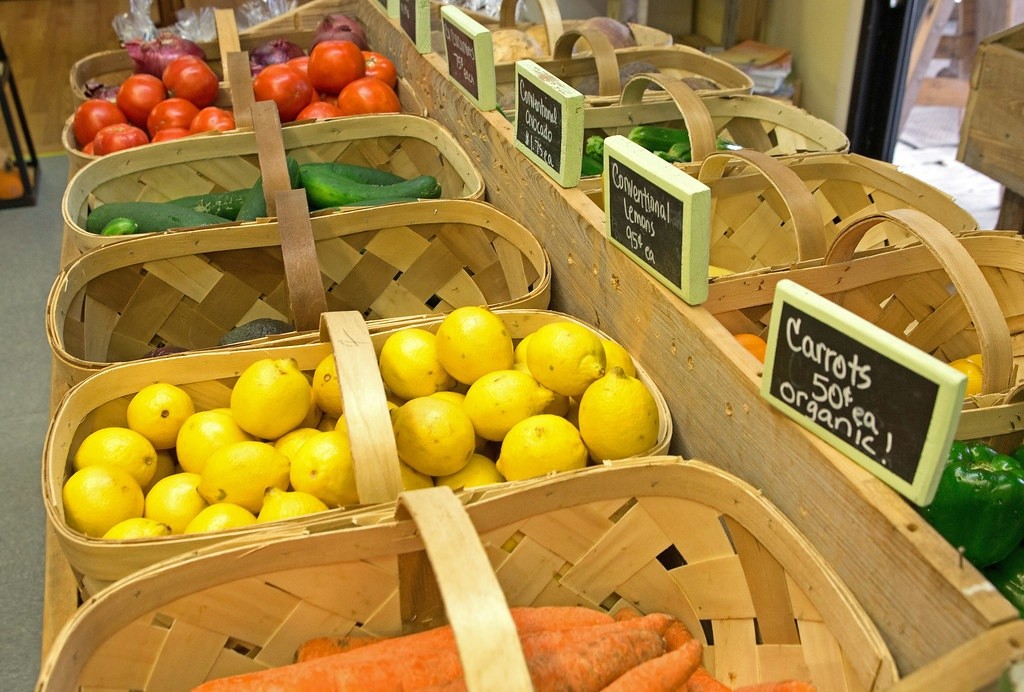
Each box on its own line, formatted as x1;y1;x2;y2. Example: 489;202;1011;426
88;13;369;102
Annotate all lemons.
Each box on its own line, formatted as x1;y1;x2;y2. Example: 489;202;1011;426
62;304;663;540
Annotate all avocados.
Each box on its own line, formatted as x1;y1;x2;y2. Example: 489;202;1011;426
142;347;188;360
216;317;293;347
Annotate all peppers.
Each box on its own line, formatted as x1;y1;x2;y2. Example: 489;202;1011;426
945;353;984;397
921;438;1024;619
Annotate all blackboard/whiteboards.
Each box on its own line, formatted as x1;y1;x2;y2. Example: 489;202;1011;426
398;0;432;56
603;129;713;309
762;275;969;508
511;58;585;189
372;0;399;19
439;5;498;112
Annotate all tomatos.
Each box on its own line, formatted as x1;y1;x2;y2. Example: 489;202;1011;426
74;38;399;156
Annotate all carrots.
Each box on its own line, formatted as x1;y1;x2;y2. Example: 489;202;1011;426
186;606;815;691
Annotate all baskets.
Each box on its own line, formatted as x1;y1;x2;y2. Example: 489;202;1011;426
698;211;1024;452
576;73;851;188
496;28;754;119
46;198;551;383
69;8;374;118
31;457;900;692
693;152;979;282
61;50;425;168
484;0;673;63
42;307;672;597
61;115;485;252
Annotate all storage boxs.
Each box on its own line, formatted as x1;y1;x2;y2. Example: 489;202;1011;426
953;21;1024;201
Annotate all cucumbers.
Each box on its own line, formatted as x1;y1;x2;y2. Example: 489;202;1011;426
580;124;738;175
86;155;442;235
